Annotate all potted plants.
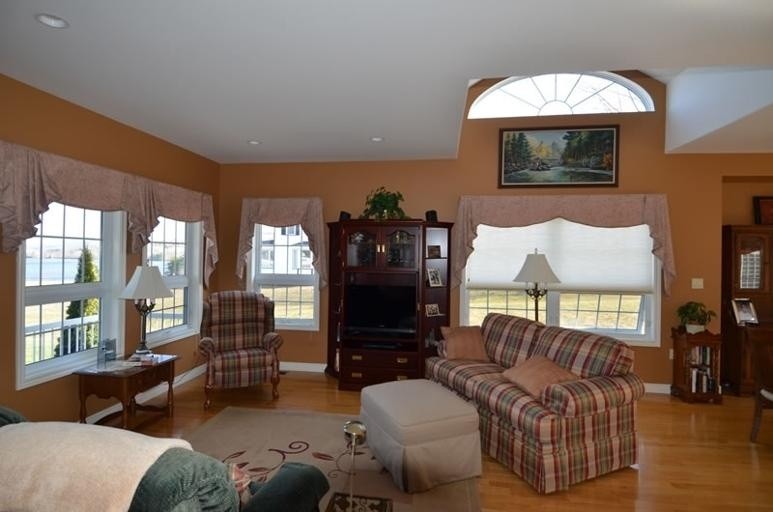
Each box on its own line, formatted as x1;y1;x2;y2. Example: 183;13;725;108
358;185;406;219
675;300;715;332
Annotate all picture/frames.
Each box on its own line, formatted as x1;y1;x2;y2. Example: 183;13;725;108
426;245;441;259
730;296;759;327
426;268;442;288
425;302;441;318
751;194;772;226
496;123;618;189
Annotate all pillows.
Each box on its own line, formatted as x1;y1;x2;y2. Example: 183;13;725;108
500;352;577;401
440;323;492;366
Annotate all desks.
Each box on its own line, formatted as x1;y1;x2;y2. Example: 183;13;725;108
73;354;179;431
323;489;393;512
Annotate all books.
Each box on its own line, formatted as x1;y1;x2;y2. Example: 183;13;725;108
124;353;158;367
690;345;713;394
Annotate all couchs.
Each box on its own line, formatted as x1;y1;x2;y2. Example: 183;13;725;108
0;415;330;511
357;377;483;494
424;306;645;494
196;287;285;410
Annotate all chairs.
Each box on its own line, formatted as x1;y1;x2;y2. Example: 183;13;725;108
747;339;772;443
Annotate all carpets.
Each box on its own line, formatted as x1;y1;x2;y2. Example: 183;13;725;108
190;403;484;512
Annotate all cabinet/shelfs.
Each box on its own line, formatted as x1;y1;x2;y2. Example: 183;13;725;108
722;222;770;398
323;220;454;393
670;324;725;405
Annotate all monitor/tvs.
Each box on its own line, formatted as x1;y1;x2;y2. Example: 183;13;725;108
345;284;416;338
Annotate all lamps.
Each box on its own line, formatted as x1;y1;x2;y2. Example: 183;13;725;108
513;246;560;321
339;417;366;512
118;264;173;353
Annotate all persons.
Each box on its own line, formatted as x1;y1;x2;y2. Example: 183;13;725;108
738;302;753;321
